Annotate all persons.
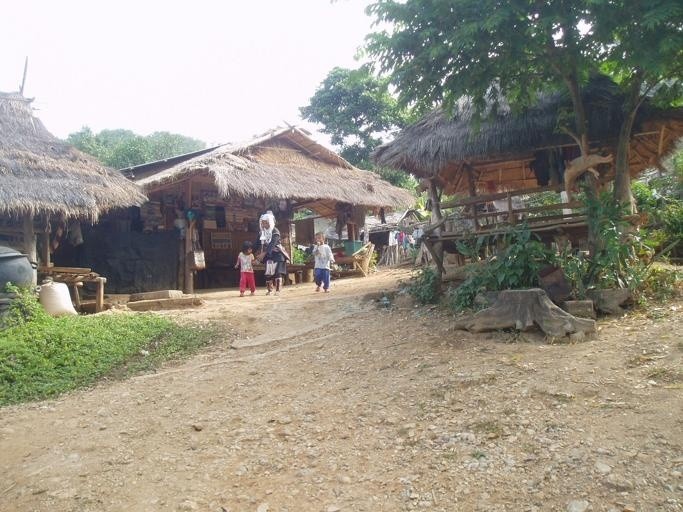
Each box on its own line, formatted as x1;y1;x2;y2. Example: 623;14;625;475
311;231;337;294
251;211;288;298
233;241;258;298
